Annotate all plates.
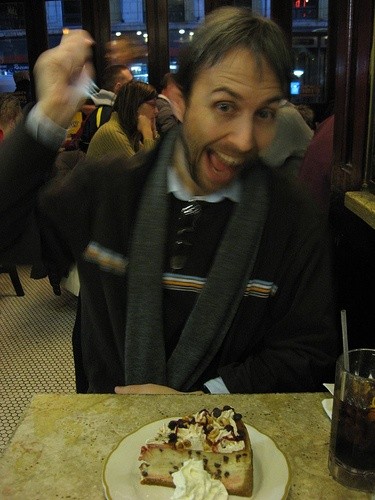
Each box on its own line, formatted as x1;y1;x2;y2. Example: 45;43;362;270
102;415;290;500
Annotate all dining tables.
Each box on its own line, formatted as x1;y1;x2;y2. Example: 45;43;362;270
0;393;375;500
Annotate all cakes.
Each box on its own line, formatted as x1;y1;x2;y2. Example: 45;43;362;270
138;405;253;500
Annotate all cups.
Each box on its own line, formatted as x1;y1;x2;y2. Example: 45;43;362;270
328;350;375;491
